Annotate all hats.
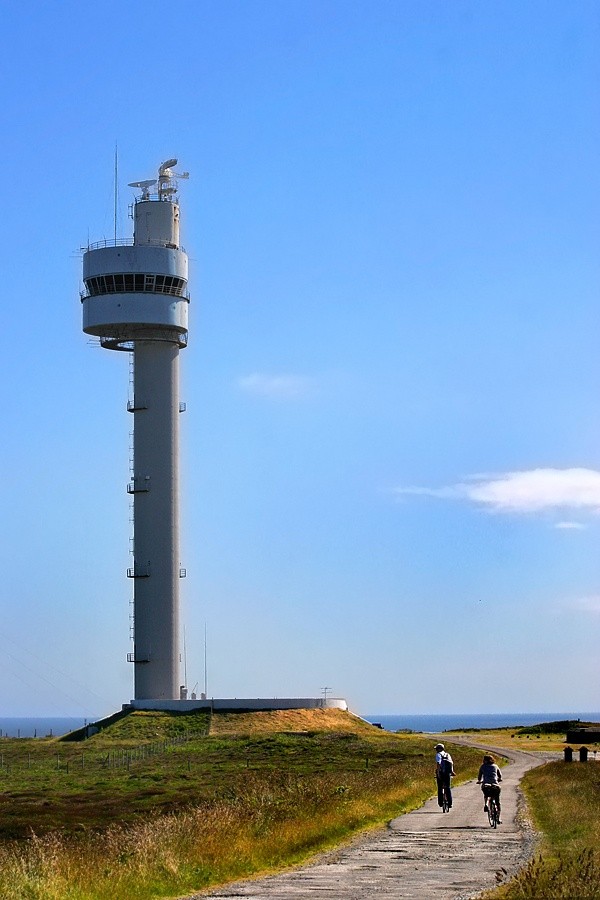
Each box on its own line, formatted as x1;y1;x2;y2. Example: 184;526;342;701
434;744;444;749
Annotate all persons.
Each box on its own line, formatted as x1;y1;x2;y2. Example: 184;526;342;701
478;755;502;815
434;743;456;808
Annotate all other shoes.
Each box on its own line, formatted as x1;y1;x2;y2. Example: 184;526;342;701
484;804;489;812
496;818;502;824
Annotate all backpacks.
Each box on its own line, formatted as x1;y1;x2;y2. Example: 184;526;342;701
437;752;452;775
